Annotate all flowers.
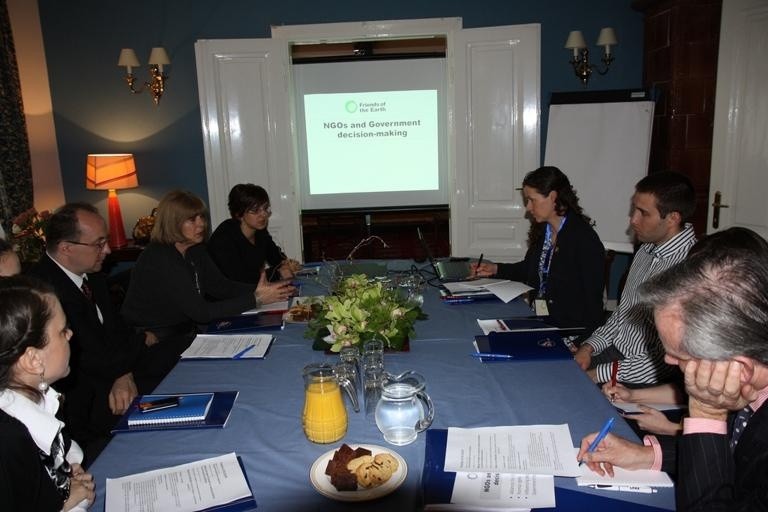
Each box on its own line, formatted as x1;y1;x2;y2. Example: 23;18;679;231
11;208;52;261
302;235;429;353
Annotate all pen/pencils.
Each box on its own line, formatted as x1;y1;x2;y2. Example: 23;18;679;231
579;417;615;467
469;353;513;359
233;345;255;359
475;253;483;275
441;298;474;303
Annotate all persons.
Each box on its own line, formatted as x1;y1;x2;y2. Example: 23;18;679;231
577;226;768;512
469;166;605;327
567;171;701;444
0;238;23;279
0;272;95;511
207;184;304;281
117;187;298;396
29;200;141;471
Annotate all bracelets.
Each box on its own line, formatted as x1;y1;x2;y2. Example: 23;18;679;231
253;291;264;309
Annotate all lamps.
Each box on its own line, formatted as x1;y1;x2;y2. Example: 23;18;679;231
85;154;140;249
118;47;171;106
564;28;618;88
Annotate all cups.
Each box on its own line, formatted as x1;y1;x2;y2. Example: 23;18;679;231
407;279;418;303
300;338;435;446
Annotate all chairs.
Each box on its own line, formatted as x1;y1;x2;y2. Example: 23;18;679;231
106;270;129;314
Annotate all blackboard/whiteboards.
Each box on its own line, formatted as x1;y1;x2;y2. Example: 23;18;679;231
544;102;655;252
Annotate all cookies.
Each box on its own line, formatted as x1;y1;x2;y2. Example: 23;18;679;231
324;444;399;493
286;303;319;322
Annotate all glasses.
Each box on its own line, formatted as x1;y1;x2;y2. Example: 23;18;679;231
57;236;110;249
248;205;271;215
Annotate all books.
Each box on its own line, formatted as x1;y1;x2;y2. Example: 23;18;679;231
126;394;214;427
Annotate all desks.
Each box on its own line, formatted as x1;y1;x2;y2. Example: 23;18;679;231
107;238;143;261
86;259;676;512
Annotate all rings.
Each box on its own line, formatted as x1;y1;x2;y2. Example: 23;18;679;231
83;479;89;489
77;471;84;482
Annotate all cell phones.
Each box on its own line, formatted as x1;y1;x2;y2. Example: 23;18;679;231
138;396;179;414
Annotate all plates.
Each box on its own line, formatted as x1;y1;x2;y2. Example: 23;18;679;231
281;309;324;324
310;444;408;502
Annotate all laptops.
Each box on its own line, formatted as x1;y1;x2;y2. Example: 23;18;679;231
416;226;479;282
